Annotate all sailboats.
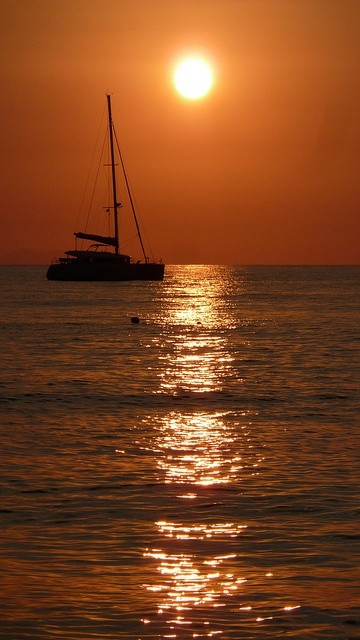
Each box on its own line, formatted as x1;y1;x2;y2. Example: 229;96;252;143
47;95;165;281
